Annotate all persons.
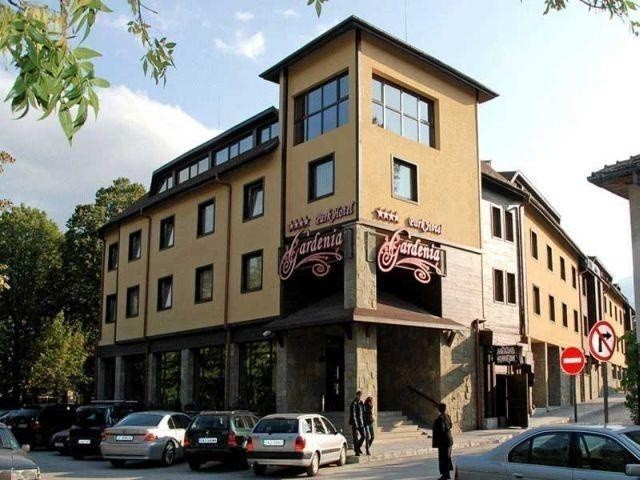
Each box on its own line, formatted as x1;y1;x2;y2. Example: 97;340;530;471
431;403;454;480
362;396;376;455
349;391;367;456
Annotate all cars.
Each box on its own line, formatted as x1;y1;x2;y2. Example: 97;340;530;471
453;424;640;480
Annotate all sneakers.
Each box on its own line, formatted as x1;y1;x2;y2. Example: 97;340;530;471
436;471;453;480
355;450;371;456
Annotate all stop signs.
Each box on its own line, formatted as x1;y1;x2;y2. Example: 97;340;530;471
560;347;586;376
